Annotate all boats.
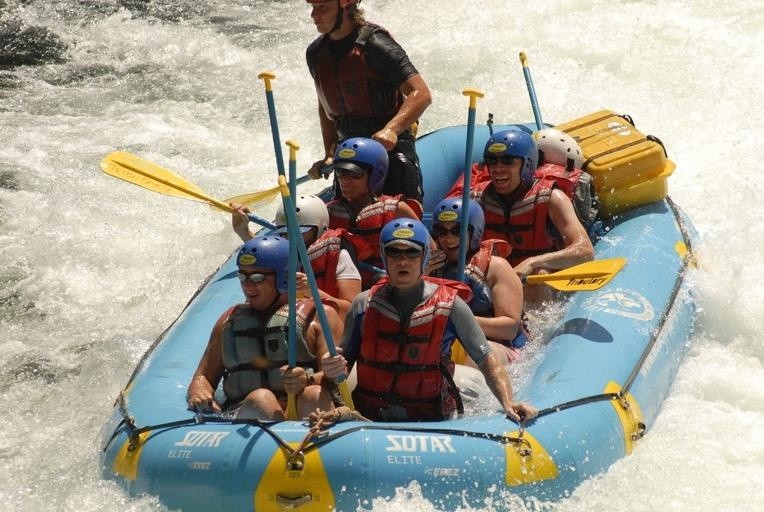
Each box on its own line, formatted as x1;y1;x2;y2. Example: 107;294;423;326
96;119;705;512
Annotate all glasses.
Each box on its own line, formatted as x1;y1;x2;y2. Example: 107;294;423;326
235;269;277;285
432;223;471;237
384;246;422;259
334;167;367;179
483;154;519;166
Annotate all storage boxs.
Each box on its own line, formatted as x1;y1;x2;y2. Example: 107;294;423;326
553;109;675;217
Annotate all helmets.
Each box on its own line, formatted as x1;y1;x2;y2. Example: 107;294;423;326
432;196;486;251
236;235;301;295
333;136;390;194
273;192;330;239
483;129;539;185
380;217;431;273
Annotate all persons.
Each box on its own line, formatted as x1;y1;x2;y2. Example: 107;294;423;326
324;137;436;290
320;216;539;424
186;234;344;421
469;129;595;309
229;194;362;328
304;0;433;205
424;196;534;370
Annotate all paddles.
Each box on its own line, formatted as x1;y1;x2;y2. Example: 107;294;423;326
100;149;277;232
221;163;334;211
450;89;484;364
521;259;628;292
